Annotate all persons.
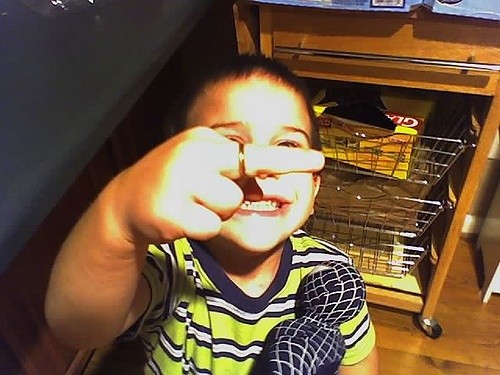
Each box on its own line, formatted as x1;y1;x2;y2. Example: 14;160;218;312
44;53;380;375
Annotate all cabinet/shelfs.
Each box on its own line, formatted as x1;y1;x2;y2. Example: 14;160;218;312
231;0;500;339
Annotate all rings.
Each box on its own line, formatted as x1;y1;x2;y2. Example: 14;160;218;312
239;142;246;178
